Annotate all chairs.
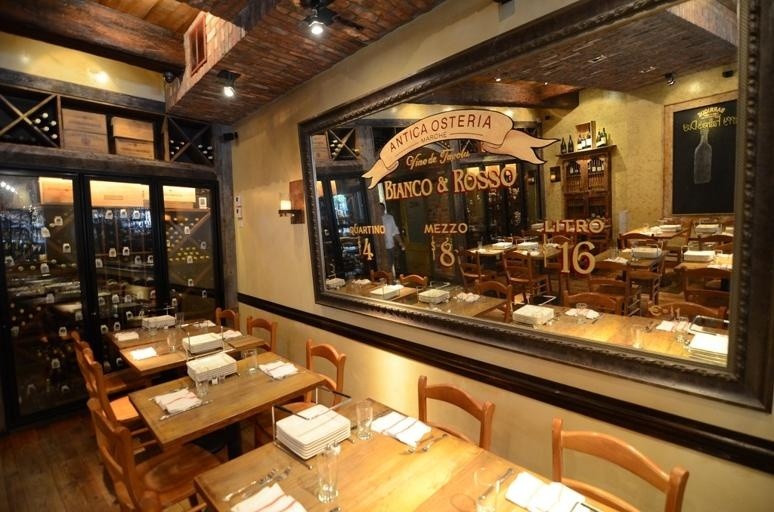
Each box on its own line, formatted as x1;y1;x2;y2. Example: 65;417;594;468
68;306;346;511
416;372;495;449
546;414;691;511
325;213;734;364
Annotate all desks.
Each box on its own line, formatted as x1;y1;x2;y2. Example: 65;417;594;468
193;395;623;512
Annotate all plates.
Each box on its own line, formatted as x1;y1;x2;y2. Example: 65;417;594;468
141;315;176;329
629;223;720;263
326;275;452;305
275;403;351;460
186;351;239;383
491;238;544;252
687;332;728;365
182;333;223;354
512;303;555;325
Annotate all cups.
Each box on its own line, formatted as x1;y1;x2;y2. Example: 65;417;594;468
168;332;177;352
629;322;645;348
198;321;209;334
672;316;688;344
312;450;341;506
576;301;587;326
176;312;184;328
472;467;500;512
194;368;208;397
532;310;544;330
354;399;374;441
241;348;259;377
146;319;159;336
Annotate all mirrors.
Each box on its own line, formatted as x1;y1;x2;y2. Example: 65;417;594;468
297;1;772;414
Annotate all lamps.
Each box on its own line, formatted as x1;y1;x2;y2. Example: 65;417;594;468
220;73;238;100
303;6;333;37
276;198;307;220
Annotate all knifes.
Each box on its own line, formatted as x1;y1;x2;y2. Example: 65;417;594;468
221;464;293;506
478;467;514;502
405;432;448;456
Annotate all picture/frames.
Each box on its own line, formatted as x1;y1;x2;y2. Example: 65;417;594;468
661;88;737;225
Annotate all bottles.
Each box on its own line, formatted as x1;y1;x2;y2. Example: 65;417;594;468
560;126;609;175
692;130;714;184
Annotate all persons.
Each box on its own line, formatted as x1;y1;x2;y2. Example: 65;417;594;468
377;201;406;275
